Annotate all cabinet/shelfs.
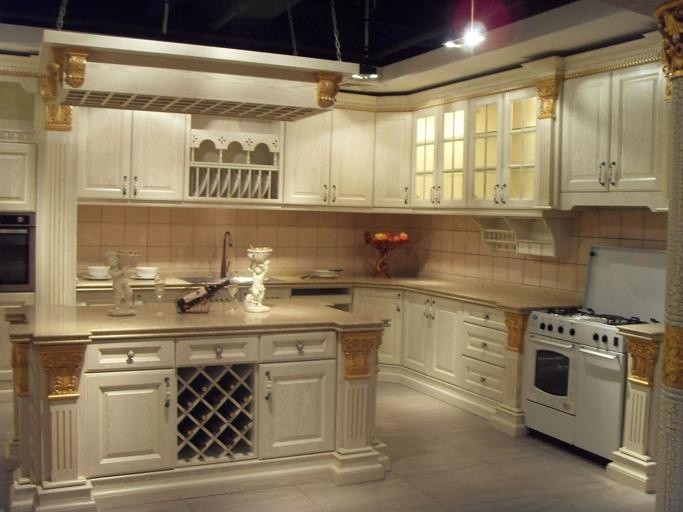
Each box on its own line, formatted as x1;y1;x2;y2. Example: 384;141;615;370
1;292;36;402
464;296;529;438
285;108;376;214
186;113;285;210
1;142;36;213
176;324;258;501
352;282;403;383
257;322;337;490
373;111;412;214
76;286;352;314
558;60;669;212
76;106;185;208
412;84;540;217
403;285;464;410
472;216;573;263
77;330;177;512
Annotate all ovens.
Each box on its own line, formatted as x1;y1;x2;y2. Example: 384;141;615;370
0;212;38;296
520;331;626;461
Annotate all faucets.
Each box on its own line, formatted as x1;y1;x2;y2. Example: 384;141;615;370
220;228;234;278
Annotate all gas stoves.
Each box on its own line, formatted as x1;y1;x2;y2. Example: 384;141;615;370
525;306;661;353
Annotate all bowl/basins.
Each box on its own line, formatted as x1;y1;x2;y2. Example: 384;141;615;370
87;266;109;277
315;269;336;275
134;267;158;277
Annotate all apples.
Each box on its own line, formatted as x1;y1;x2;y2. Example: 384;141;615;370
373;231;409;241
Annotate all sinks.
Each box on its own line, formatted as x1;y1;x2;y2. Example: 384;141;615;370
177;274;230;285
231;274;284;284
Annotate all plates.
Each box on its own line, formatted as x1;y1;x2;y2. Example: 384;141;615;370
310;273;338;278
82;275;112;280
130;274;160;280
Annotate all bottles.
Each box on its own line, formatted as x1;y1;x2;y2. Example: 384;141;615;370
176;279;230;312
